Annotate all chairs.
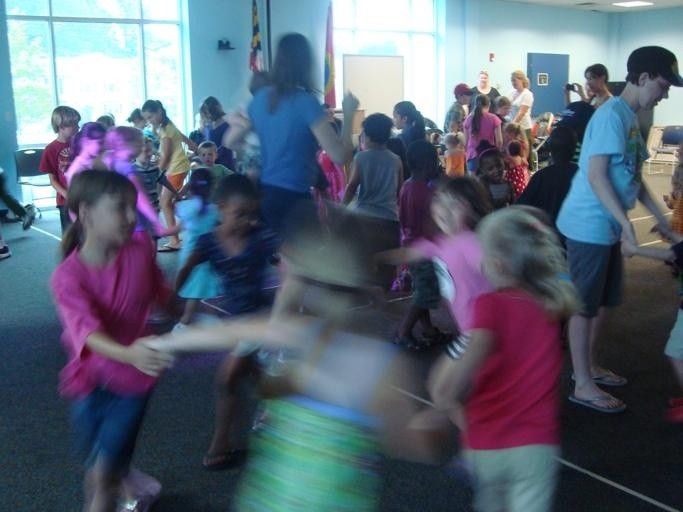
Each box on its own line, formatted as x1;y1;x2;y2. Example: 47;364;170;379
644;126;683;176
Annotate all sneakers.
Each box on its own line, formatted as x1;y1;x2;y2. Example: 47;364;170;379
0;244;11;259
24;215;34;229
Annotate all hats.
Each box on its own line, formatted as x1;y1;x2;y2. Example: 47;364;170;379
627;46;683;87
454;84;470;95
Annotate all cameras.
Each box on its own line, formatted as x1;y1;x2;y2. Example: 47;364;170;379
567;84;574;90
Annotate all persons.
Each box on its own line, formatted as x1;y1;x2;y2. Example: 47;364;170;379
0;164;39;260
36;32;682;512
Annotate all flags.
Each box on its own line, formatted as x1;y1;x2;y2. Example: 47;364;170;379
323;3;336;110
247;0;264;76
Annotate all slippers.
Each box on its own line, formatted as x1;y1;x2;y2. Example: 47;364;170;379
203;448;235;470
568;393;627;413
158;245;181;252
572;370;627;386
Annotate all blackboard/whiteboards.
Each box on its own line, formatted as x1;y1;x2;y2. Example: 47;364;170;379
343;54;405;122
